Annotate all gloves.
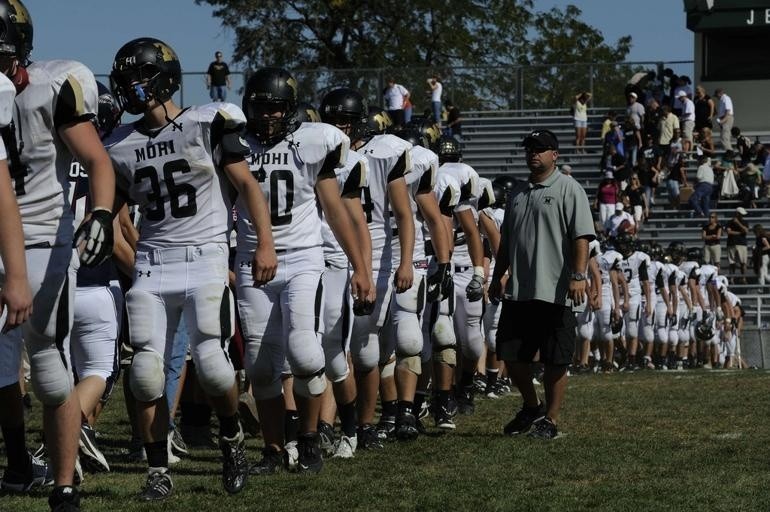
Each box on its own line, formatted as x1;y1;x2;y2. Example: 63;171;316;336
609;309;624;334
466;274;486;302
71;209;114;271
426;262;453;305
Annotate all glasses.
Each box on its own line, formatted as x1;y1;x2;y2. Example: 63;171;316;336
630;178;636;180
525;146;555;153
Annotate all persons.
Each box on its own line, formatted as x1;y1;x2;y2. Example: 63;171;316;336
572;232;745;375
595;68;770;233
571;90;592;154
754;224;770;293
487;130;596;440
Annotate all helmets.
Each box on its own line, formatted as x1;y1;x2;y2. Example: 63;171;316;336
651;244;663;261
491;183;507;208
403;118;438;154
108;37;182;115
693;321;714;341
597;232;610;253
317;88;369;145
494;175;516;209
394;128;421;148
241;67;297;143
297;101;321;123
367;103;392;134
686;248;703;267
669;240;686;266
611;232;635;259
0;0;34;70
430;134;462;164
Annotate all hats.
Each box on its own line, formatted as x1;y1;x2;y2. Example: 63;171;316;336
520;130;558;147
615;201;624;211
674;90;687;99
604;170;614;179
627;92;638;99
736;207;748;215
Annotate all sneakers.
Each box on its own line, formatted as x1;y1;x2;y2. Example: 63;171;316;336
48;486;80;512
166;429;181;463
395;413;419;442
294;430;325;475
332;433;358;459
434;397;457;429
218;421;249;494
29;451;49;478
473;369;487;393
503;399;545;437
2;468;46;493
532;366;545;383
78;422;110;474
457;384;476;415
120;445;147;463
172;430;189;455
620;358;636;371
653;361;666;370
357;423;384;449
134;466;174;501
486;368;501;399
576;363;590;374
495;378;513;395
73;454;85;487
375;412;395;439
640;358;655;370
527;416;555;439
600;362;614;374
319;420;339;459
668;359;677;370
249;441;289;477
415;403;428;419
